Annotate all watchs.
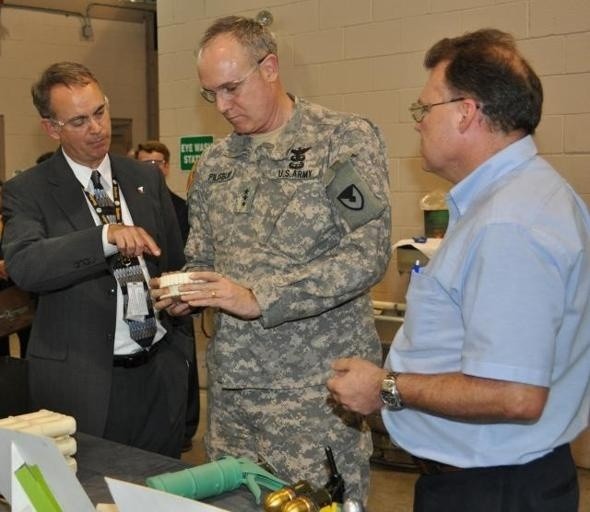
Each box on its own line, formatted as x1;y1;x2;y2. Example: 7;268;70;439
379;368;405;411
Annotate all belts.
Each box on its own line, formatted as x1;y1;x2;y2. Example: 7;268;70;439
114;351;149;368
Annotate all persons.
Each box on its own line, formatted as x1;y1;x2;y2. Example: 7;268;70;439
144;13;394;511
0;181;39;360
327;28;587;511
0;61;191;460
136;141;192;250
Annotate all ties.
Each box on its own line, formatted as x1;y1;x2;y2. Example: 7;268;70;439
91;171;156;350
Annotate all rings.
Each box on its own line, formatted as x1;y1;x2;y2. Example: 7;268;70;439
208;288;217;297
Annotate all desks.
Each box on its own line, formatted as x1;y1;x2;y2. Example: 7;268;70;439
52;429;267;510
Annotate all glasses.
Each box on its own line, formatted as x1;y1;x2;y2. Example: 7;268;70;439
410;97;467;123
200;52;269;103
51;96;109;129
